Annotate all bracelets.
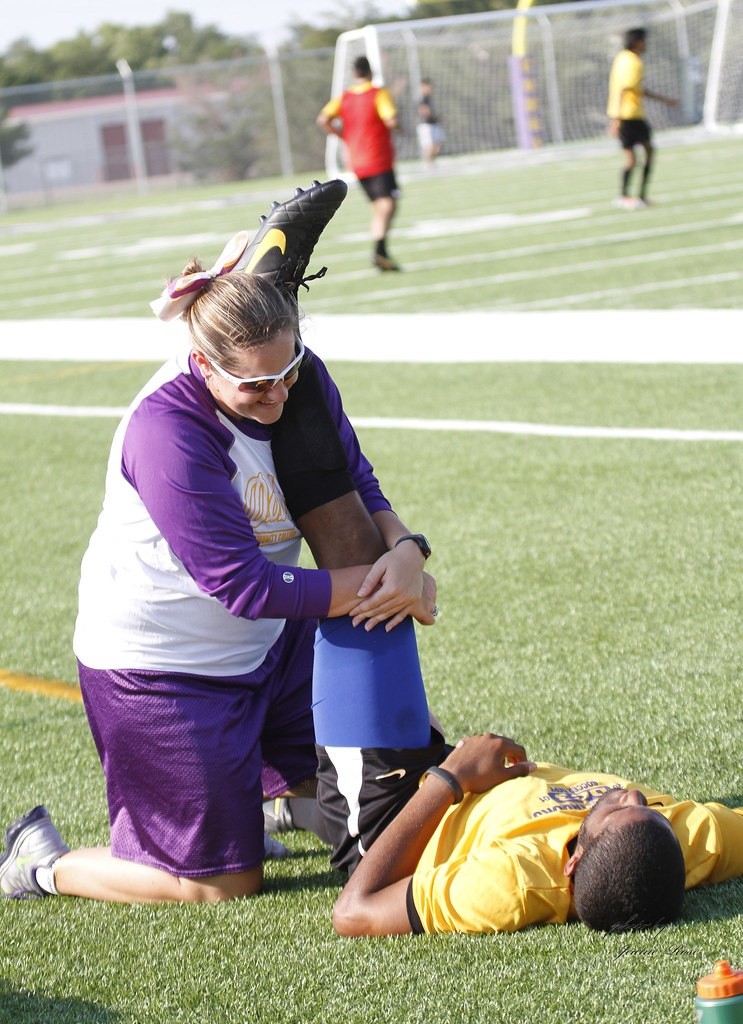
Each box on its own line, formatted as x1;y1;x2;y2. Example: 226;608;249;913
424;766;464;805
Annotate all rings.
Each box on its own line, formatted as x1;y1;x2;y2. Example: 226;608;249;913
433;606;438;616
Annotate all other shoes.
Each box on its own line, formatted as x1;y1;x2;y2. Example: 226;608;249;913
373;252;400;270
619;197;651;209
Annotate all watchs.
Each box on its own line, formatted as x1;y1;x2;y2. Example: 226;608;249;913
394;533;432;560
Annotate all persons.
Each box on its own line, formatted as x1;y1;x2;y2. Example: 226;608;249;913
1;257;438;911
416;77;444;180
226;179;743;938
606;27;683;209
315;56;404;270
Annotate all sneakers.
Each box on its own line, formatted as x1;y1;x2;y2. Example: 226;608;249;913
0;805;68;897
232;180;346;310
261;788;294;834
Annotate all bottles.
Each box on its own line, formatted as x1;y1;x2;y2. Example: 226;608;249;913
694;959;743;1024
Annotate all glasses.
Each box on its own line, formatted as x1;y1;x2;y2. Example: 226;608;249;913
203;332;305;393
